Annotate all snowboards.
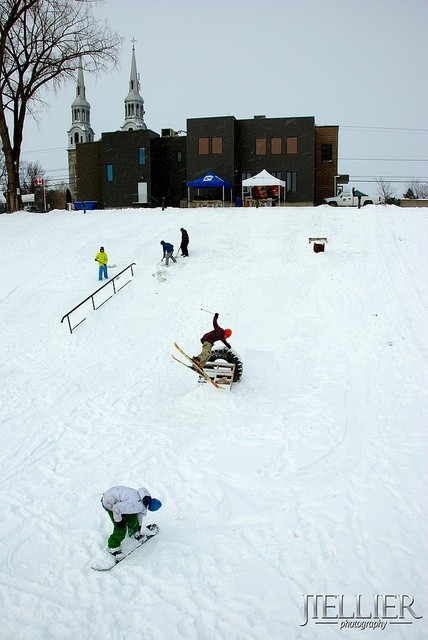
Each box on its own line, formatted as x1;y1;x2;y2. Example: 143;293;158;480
91;523;160;571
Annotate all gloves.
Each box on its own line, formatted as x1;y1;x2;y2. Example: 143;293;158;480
215;313;218;317
117;519;125;528
229;346;231;349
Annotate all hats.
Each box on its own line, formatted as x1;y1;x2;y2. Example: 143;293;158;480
225;329;231;337
148;499;161;511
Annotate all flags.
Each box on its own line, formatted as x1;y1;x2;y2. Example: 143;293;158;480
34;178;44;185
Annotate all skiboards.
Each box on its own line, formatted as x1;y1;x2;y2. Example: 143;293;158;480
171;342;226;389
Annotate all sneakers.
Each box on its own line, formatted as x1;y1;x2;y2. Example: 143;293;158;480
192;355;206;373
108;544;123;560
131;524;157;543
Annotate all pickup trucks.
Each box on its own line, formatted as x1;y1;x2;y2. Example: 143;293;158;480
322;191;379;208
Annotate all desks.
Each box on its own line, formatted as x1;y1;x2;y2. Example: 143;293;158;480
193;200;222;207
247;199;276;207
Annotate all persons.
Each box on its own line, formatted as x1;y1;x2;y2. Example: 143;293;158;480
100;486;162;559
180;228;189;257
192;312;232;370
160;240;177;265
95;246;108;281
